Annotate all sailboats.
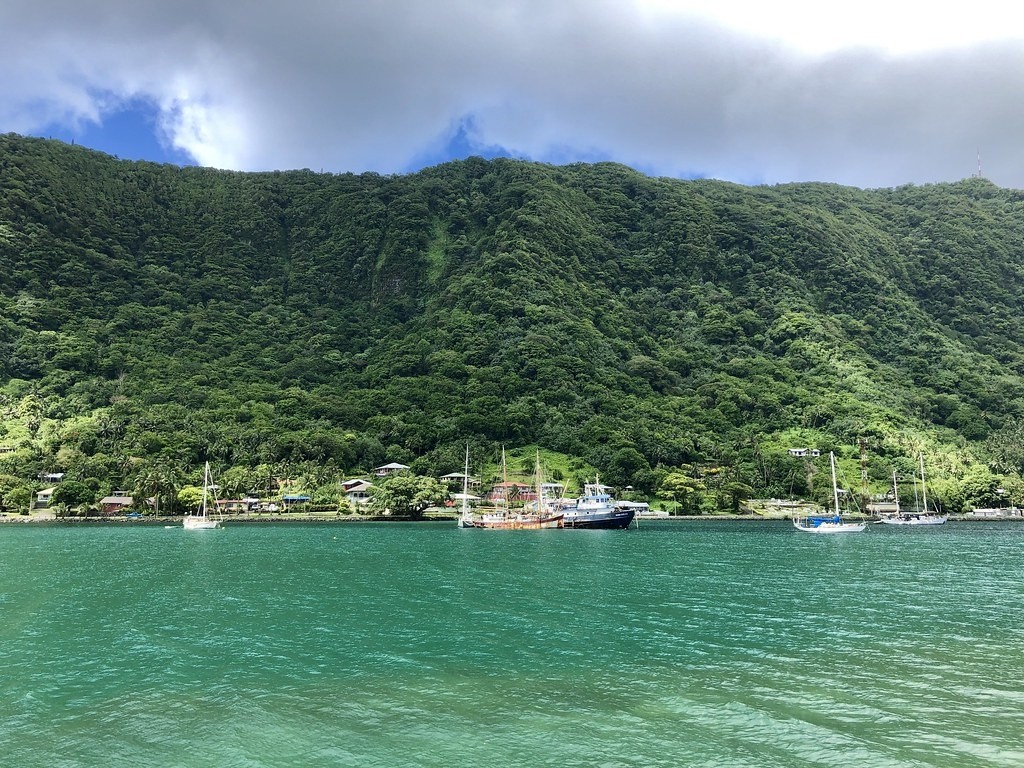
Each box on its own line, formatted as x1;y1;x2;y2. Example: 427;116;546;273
879;452;950;526
792;450;868;533
182;460;224;530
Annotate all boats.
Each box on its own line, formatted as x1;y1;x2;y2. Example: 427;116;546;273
457;443;635;531
